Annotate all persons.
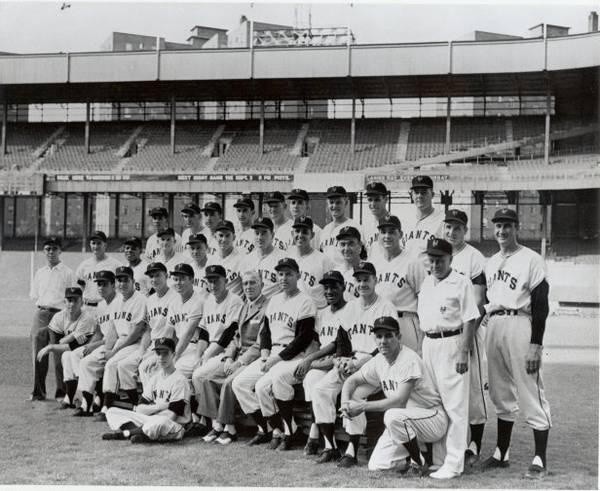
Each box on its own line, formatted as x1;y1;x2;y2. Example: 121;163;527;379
27;175;550;481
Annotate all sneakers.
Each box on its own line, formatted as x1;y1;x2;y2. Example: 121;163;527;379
529;460;546;474
102;433;122;441
185;423;358;468
28;393;46;401
130;435;149;444
56;396;108;421
479;456;510;469
404;450;480;480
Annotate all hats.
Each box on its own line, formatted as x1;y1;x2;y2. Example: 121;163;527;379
427;238;453;256
377;216;401;227
95;262;224;280
149;185;359;245
65;287;83;298
443;209;467;224
318;271;343;284
364;182;386;195
124;237;143;248
370;317;400;332
274;258;297;271
352;262;376;276
153;337;175;351
492;208;518;222
410;176;433;189
43;235;61;248
88;231;106;243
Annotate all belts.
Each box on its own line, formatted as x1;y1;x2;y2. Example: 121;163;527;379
425;330;459;338
38;306;57;313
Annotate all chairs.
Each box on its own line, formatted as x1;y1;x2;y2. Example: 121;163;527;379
0;114;599;181
